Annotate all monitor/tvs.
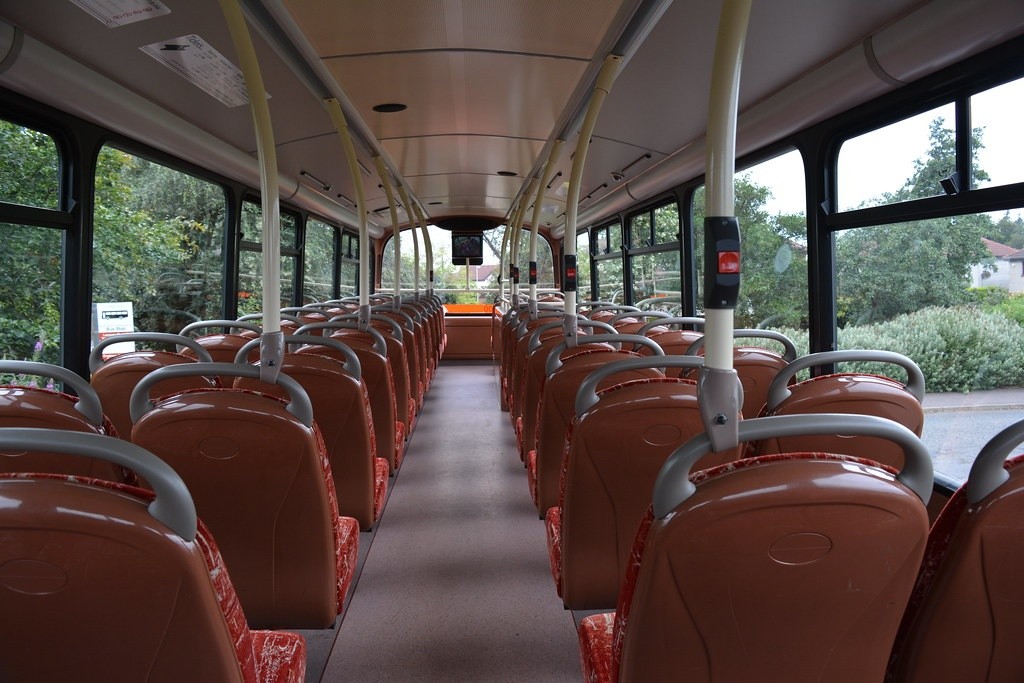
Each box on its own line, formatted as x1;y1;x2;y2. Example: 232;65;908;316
452;234;483;259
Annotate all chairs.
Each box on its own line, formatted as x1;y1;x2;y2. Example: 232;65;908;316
490;288;1024;683
1;286;449;683
